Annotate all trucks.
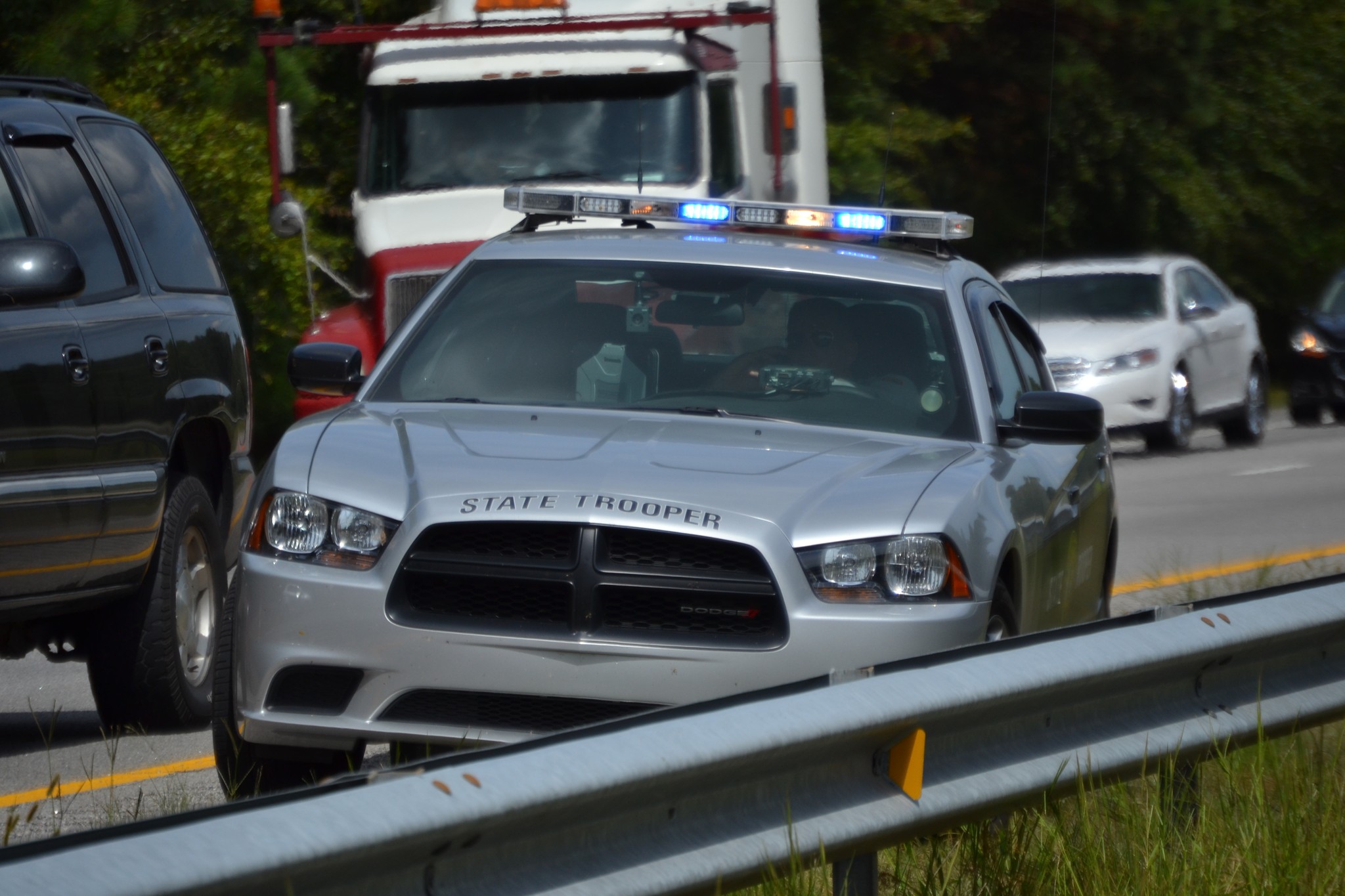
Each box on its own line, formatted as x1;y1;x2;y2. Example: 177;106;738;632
254;3;873;438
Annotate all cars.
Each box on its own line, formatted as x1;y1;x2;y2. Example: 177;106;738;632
1286;270;1345;430
1001;251;1270;455
209;180;1122;808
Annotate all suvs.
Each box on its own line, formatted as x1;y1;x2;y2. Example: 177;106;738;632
0;76;259;736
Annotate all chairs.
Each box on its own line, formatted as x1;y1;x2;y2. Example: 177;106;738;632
850;303;931;381
